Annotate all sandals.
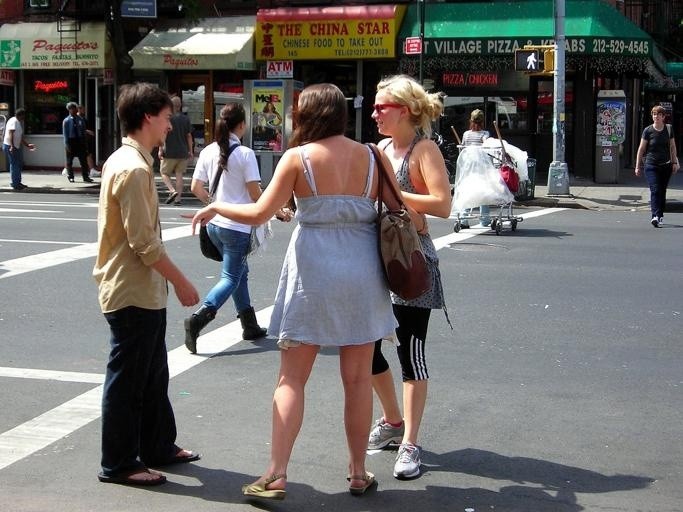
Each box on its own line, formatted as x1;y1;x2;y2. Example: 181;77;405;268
242;474;288;499
347;471;376;494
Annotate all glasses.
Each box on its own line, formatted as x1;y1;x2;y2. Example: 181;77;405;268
372;103;401;114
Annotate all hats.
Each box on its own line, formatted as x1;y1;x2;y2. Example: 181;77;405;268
470;109;485;123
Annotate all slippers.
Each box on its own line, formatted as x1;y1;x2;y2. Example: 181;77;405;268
165;192;179;204
143;447;200;466
174;201;184;206
98;465;166;485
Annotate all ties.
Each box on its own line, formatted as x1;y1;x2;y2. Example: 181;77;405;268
73;118;78;136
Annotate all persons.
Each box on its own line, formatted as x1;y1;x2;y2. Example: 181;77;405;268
92;82;200;486
2;108;36;190
256;102;282;141
367;74;453;481
157;96;195;206
636;106;679;229
180;83;375;501
460;108;492;230
185;102;268;354
63;101;102;183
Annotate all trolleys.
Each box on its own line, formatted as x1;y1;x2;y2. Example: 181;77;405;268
451;123;542;238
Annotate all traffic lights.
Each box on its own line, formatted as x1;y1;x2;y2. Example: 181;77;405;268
512;49;546;74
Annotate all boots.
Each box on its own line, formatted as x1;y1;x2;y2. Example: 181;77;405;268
237;306;268;340
184;304;215;354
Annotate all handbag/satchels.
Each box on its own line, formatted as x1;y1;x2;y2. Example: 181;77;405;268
365;144;432;301
500;164;520;193
200;222;223;262
669;153;680;175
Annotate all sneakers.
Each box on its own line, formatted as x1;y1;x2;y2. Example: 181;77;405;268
650;217;658;227
393;444;422;479
90;168;101;176
461;224;470;229
658;217;663;225
368;416;404;450
13;184;27;190
62;168;69;177
